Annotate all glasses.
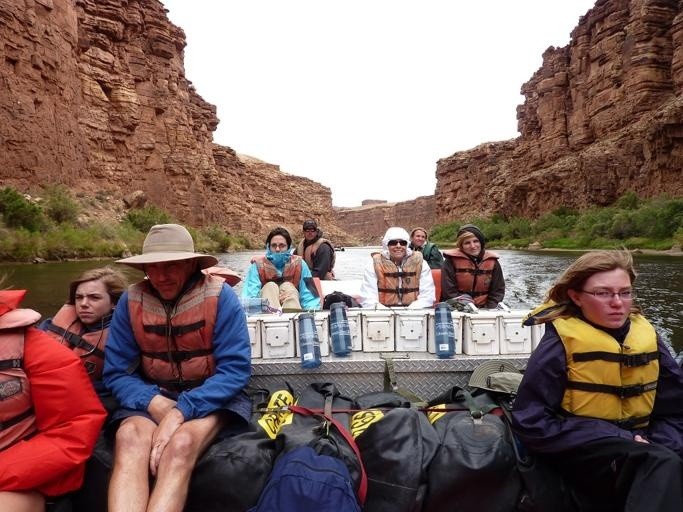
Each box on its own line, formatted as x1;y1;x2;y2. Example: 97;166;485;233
580;288;638;302
304;229;316;232
270;243;286;248
388;240;407;246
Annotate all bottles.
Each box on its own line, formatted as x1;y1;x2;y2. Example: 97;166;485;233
433;301;455;357
329;302;352;356
238;297;269;316
297;313;321;369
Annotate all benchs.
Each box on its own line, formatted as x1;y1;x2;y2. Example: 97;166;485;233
429;268;442;306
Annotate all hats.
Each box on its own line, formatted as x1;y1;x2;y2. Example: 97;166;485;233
114;223;219;271
303;220;317;228
381;227;412;259
468;361;521;393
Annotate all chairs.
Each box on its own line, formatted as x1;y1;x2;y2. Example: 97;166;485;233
313;276;324;309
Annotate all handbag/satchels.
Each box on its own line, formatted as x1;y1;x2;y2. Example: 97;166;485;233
323;291;362;310
184;380;569;512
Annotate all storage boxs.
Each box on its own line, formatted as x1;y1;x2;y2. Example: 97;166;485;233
243;305;545;359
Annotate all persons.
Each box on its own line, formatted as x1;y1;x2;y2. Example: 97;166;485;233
0;289;109;512
408;227;444;269
241;228;322;312
101;223;252;512
357;227;436;310
37;266;127;384
292;220;336;280
439;224;505;309
510;245;683;512
